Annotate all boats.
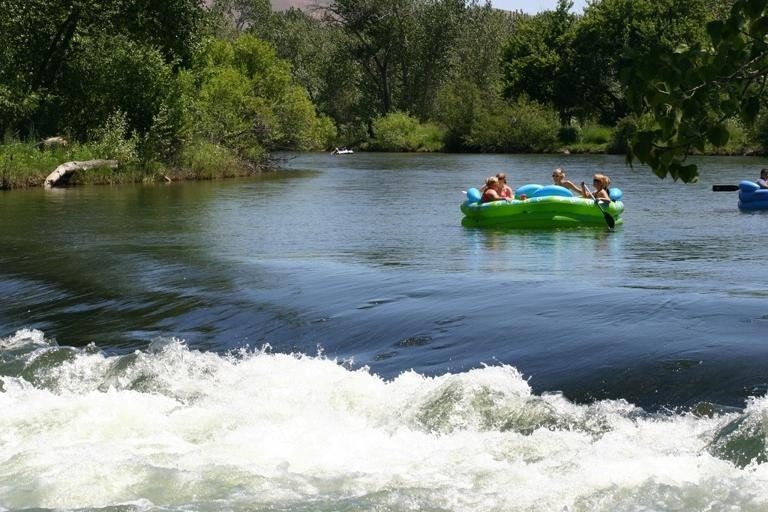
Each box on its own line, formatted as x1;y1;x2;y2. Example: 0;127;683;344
331;148;354;154
460;184;624;230
737;179;768;211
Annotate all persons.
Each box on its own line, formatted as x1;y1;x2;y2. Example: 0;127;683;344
336;147;347;152
756;169;768;189
552;168;611;203
480;173;514;203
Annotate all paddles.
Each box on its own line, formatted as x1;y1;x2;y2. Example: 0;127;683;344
581;181;615;229
712;185;740;192
